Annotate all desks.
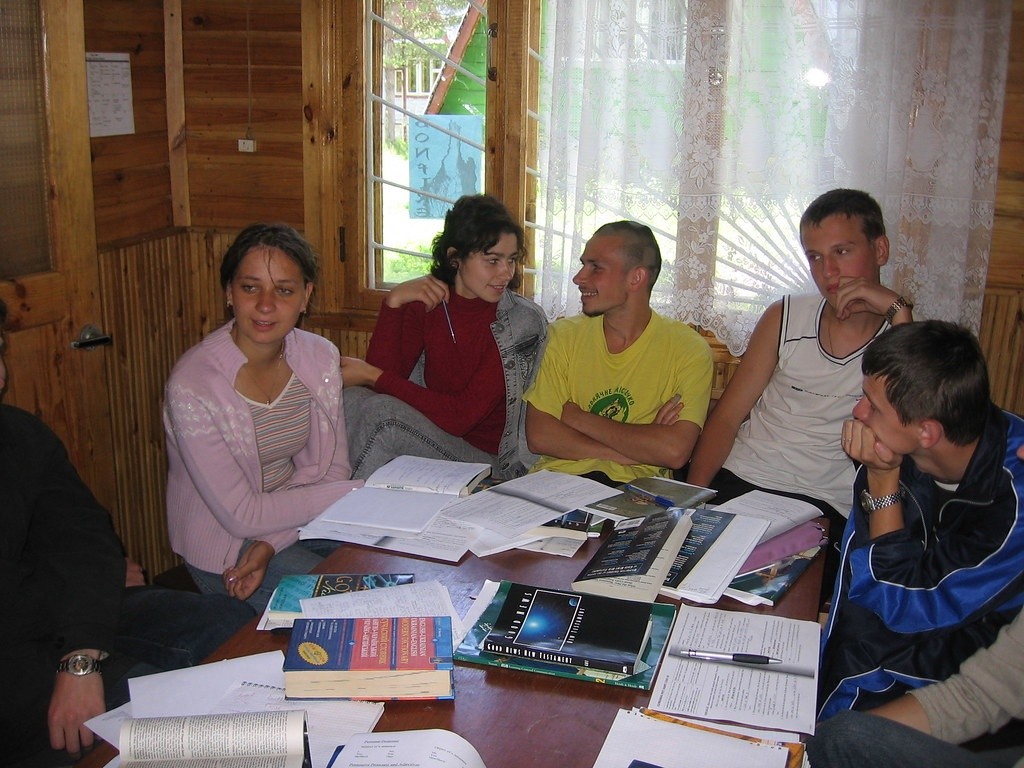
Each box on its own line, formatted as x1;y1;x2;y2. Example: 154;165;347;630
71;517;831;768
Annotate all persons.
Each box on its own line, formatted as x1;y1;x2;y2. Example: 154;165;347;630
804;439;1024;768
0;300;258;768
522;221;713;490
816;318;1024;723
340;194;547;480
689;189;930;610
164;220;366;618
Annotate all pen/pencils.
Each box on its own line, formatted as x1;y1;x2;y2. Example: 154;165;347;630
624;484;674;508
439;297;461;344
373;483;438;496
680;648;782;671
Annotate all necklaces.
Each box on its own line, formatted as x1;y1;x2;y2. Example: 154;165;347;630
828;312;835;357
246;337;284;406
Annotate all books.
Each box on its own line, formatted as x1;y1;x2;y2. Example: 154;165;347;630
112;453;826;768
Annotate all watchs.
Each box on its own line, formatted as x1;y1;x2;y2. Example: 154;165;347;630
56;654;103;676
885;296;914;323
861;489;901;512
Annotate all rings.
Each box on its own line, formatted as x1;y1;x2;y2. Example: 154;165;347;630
843;438;852;442
